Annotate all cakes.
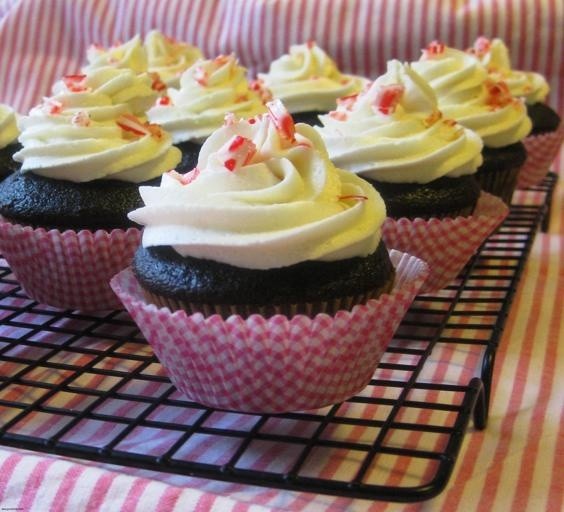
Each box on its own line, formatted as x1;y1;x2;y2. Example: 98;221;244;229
0;26;561;318
